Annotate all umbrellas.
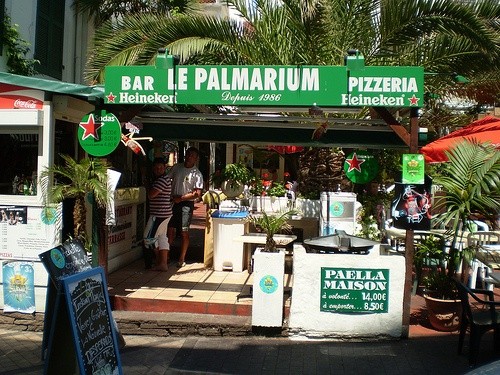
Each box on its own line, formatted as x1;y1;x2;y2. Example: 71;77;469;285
419;116;500;163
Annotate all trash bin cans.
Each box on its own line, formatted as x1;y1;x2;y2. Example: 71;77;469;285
210;210;248;272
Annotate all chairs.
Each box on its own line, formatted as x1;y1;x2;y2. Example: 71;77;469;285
396;221;500;368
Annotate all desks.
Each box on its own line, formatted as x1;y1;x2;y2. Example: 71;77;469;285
248;214;319;257
236;233;296;301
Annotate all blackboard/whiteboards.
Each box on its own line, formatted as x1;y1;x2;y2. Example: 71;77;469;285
39;237;127;352
44;266;123;375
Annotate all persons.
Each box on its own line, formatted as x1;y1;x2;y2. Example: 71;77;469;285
143;157;173;271
168;147;203;268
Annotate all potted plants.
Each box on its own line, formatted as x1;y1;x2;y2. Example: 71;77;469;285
417;151;500;332
250;210;293;328
217;162;252;200
